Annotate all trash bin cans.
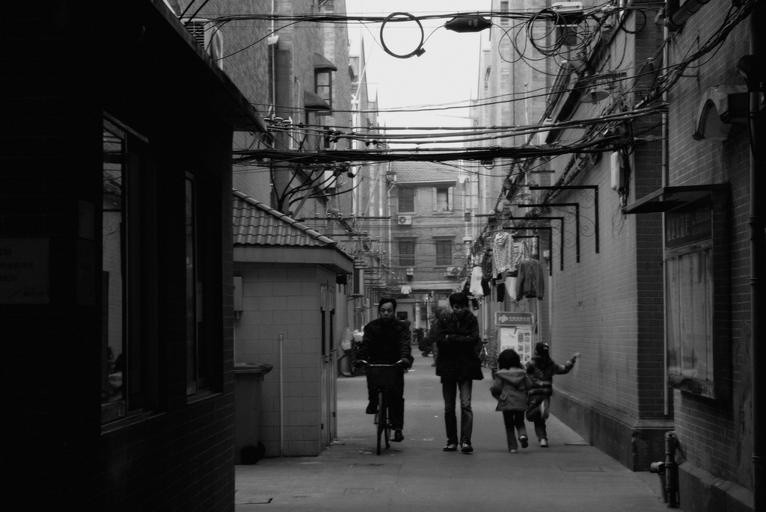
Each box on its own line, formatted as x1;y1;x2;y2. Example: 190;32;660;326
233;364;274;465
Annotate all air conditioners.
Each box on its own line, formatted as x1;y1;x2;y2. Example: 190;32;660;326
397;215;413;225
446;266;461;277
181;16;224;67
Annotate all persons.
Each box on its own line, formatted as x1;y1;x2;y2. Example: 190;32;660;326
525;342;581;448
432;293;484;453
490;348;534;453
410;326;432;356
353;297;415;442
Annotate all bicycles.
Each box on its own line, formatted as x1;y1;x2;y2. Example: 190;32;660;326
337;334;360;377
480;341;498;369
353;360;411;455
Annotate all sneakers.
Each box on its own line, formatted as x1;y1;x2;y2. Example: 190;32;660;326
540;400;549;419
366;400;378;414
510;435;529;453
394;430;404;443
540;438;547;447
443;442;474;453
